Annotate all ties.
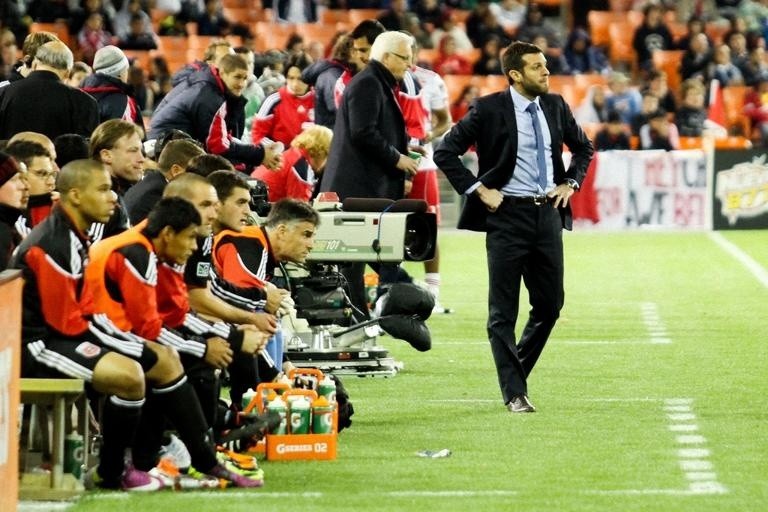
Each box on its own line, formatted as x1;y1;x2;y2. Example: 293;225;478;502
525;103;549;193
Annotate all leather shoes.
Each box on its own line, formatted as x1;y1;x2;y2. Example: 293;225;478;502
504;394;535;413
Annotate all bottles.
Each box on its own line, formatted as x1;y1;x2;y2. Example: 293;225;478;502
332;286;344;306
367;279;378;306
64;428;85;479
241;369;337;436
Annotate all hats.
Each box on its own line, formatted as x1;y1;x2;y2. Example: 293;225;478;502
90;43;129;74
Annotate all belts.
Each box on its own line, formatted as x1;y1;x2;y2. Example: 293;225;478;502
504;195;557;206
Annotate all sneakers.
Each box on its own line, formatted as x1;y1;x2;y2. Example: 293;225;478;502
83;408;267;492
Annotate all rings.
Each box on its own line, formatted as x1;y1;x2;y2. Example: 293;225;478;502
557;194;563;199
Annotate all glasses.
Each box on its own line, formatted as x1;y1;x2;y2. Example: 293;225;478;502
389;51;411;61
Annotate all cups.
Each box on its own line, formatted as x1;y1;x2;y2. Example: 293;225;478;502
404;151;422;181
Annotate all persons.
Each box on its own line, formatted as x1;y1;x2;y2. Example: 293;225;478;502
434;41;595;412
0;126;333;493
0;1;767;154
320;31;426;320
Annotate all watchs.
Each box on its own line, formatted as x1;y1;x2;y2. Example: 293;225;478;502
563;179;579;192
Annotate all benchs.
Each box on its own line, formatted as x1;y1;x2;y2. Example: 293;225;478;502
13;372;93;491
